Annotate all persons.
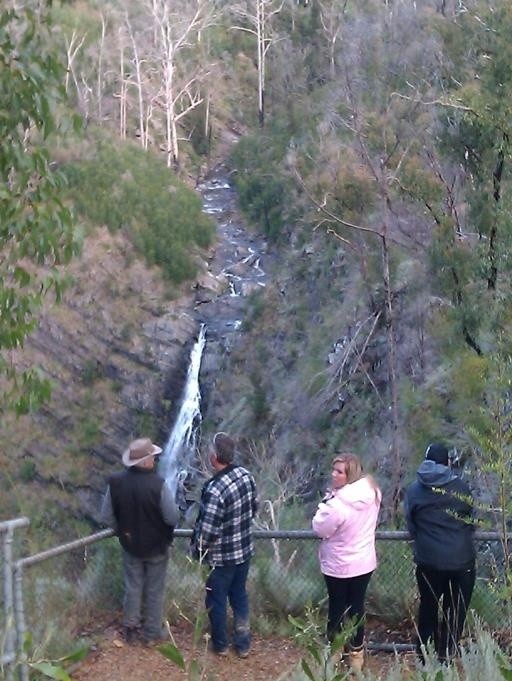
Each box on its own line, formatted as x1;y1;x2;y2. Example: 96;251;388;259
404;442;476;670
184;432;258;660
311;452;381;672
99;438;180;648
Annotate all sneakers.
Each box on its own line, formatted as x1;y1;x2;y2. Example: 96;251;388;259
144;627;169;648
125;628;139;645
218;647;230;656
236;648;248;658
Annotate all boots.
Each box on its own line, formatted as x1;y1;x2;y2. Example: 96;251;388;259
347;649;365;674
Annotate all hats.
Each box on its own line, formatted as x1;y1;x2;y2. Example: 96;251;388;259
424;442;449;464
121;437;163;468
